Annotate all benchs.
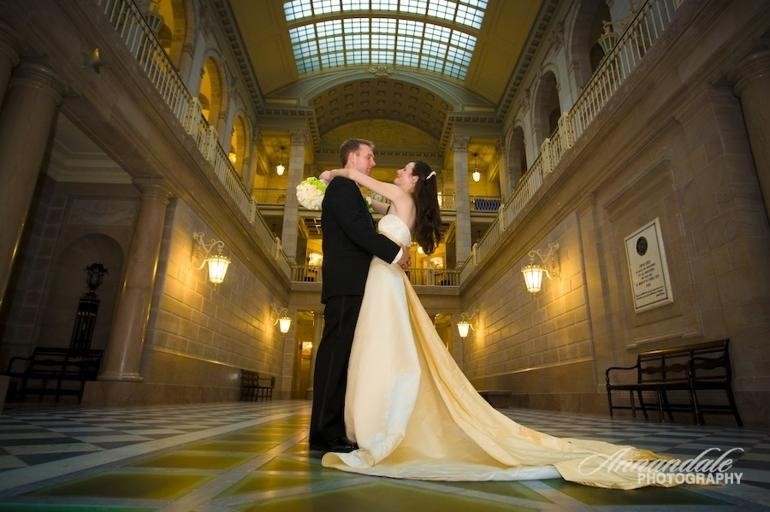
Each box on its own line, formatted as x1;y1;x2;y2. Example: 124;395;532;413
477;388;512;410
9;345;104;404
240;370;276;400
602;338;740;425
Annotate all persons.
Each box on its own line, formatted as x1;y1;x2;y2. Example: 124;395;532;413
317;159;727;491
308;136;413;455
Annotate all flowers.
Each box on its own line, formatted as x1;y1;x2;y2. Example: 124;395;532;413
296;174;375;220
364;197;375;214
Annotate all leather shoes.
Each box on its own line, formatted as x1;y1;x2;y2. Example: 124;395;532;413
310;436;358;453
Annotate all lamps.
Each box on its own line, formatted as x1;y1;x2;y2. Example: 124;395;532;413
68;260;107;345
190;233;231;285
521;241;562;295
275;144;287;177
595;10;633;60
142;1;166;36
269;302;292;337
458;310;479;340
468;152;482;182
300;340;313;355
